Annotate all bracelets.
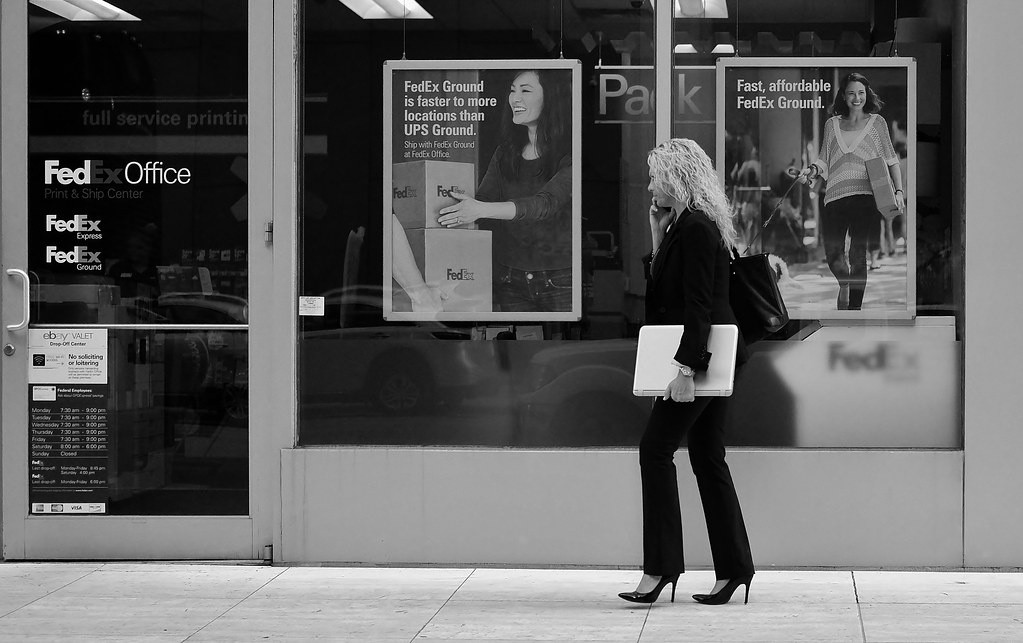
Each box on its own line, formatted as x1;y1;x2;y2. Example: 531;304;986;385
895;189;903;195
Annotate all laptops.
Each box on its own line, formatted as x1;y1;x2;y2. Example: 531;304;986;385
633;325;738;397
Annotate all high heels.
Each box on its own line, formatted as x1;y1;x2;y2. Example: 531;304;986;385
691;574;753;605
618;572;680;603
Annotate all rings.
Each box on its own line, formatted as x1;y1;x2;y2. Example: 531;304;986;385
456;217;460;224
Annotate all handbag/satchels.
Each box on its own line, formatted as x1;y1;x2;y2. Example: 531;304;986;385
728;242;790;346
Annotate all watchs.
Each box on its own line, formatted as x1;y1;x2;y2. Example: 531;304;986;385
678;365;696;377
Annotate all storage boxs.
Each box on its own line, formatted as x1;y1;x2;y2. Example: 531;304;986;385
393;160;494;313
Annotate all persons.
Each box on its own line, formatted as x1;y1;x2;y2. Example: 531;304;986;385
798;72;904;310
391;69;572;313
617;138;756;605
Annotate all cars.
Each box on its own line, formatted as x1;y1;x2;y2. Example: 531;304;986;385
123;284;494;427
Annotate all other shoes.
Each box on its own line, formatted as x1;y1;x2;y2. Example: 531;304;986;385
837;287;849;309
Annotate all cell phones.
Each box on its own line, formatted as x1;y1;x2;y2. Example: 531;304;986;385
655;200;671;212
